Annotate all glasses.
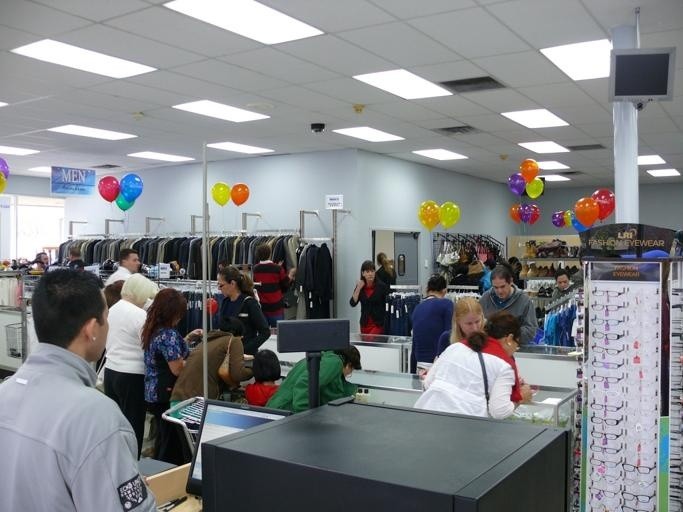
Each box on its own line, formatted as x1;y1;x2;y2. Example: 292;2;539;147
589;487;620;499
592;360;623;369
591;375;623;384
591;389;622;398
591;305;623;311
591;431;621;440
622;478;654;489
512;337;521;351
590;458;621;468
591;417;622;426
591;403;622;412
592;332;623;340
590;319;623;325
621;505;655;512
621;491;654;503
592;345;623;355
622;463;656;474
589;472;620;484
590;443;622;454
217;281;228;288
592;291;622;297
346;370;352;378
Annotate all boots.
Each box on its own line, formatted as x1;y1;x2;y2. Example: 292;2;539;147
522;242;530;259
529;240;536;259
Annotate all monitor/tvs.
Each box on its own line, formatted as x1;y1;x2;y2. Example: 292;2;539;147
186;398;292;499
607;46;676;112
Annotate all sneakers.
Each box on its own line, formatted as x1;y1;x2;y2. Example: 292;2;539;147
546;288;554;297
547;263;555;276
520;264;529;276
534;307;544;318
538;286;546;297
536;266;548;277
527;264;538;277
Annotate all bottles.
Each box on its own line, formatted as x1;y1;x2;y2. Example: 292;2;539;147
356;389;364;402
363;389;369;402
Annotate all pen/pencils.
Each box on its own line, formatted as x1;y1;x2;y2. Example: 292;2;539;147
158;498;179;509
162;496;187;512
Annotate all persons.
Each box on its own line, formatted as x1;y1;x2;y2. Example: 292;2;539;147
570;249;582;284
349;260;387;341
37;252;49;267
103;248;281;462
412;265;539;419
265;343;362;413
449;248;527;294
67;248;87;268
376;252;397;293
1;268;158;512
273;255;298;320
551;268;575;302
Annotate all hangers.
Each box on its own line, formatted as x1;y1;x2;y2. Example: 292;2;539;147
385;288;481;301
65;232;297;241
548;286;578;322
157;278;223;294
442;235;503;256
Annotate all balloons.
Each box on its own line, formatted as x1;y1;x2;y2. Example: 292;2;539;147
508;159;615;233
211;182;231;207
230;183;249;206
98;173;143;212
0;158;10;195
418;200;461;232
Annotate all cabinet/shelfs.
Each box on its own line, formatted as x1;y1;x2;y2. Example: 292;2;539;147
0;264;185;381
260;326;412;373
201;397;571;511
519;242;581;328
403;330;576;389
241;360;580;429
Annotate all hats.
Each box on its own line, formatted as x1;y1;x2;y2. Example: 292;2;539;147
342;346;361;370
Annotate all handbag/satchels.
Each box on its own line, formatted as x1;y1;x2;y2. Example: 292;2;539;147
445;252;460;266
468;261;484;275
475;238;487;263
460;240;468;265
466;243;479;264
217;352;240;392
484;244;493;263
436;240;447;266
